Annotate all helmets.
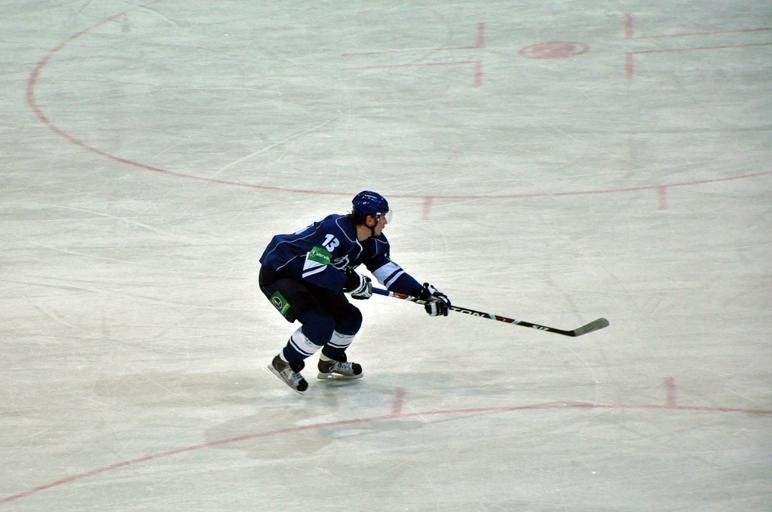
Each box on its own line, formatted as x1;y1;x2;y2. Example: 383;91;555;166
351;190;390;216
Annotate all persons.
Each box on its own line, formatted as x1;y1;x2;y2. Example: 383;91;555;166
257;190;453;392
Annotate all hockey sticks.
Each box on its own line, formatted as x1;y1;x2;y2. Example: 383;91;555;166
371;286;610;338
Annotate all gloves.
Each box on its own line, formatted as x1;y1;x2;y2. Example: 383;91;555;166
417;282;451;317
342;267;373;300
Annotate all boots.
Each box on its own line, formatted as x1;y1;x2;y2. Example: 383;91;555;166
318;349;362;376
272;347;308;391
